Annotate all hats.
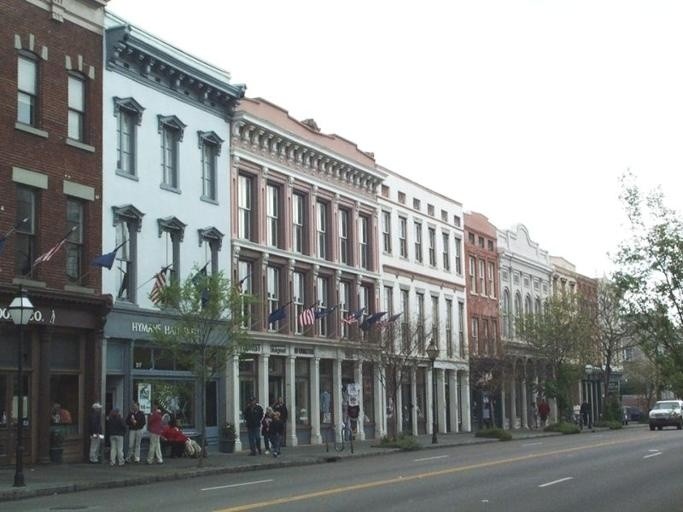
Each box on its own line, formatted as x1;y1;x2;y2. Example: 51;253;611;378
92;402;103;409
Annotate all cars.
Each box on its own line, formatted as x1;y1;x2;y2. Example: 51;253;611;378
616;406;644;422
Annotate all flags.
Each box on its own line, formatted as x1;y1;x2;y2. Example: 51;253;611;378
30;233;244;314
267;301;399;335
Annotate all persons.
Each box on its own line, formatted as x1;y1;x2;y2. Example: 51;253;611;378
243;396;288;457
347;397;360;434
47;397;72;425
579;400;589;427
526;402;538;429
539;398;551;427
86;400;187;467
471;400;497;433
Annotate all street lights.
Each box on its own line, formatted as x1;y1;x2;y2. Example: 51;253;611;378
426;337;439;444
7;282;34;487
584;360;592;429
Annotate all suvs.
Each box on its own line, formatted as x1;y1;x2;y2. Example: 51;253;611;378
648;399;683;430
